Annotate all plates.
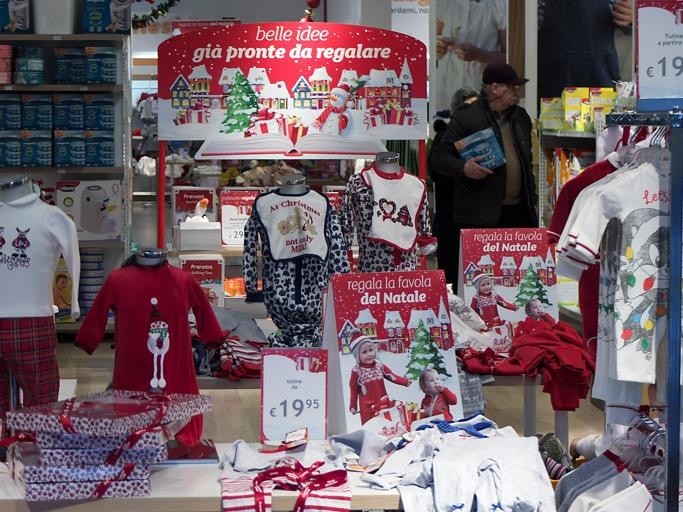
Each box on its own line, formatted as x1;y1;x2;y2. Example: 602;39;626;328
538;130;605;345
0;1;130;346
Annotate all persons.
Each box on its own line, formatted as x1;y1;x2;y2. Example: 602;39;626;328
431;61;538;227
335;147;443;279
418;369;457;422
467;270;519;328
236;172;357;352
346;328;411;426
537;1;633;106
0;171;82;446
432;1;507;110
523;300;556;323
75;246;227;449
425;86;479;295
51;273;69;307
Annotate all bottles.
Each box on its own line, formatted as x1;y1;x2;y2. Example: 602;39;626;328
472;269;489;285
483;64;529;86
348;329;370;352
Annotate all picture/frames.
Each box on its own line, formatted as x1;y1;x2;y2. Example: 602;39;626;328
191;315;571;454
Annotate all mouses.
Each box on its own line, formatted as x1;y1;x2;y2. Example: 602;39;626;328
582;111;671;189
